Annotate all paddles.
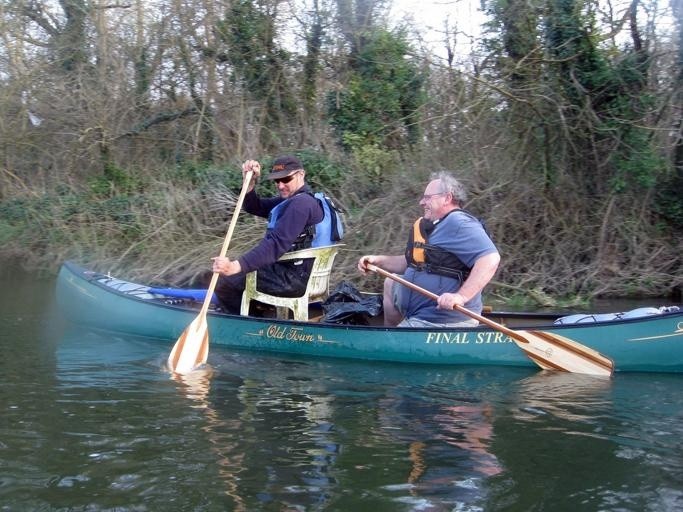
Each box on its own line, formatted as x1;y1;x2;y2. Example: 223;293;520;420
363;260;613;379
169;169;254;374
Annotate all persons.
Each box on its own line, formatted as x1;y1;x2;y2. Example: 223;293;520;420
396;383;500;512
358;176;501;329
251;419;348;512
211;156;344;319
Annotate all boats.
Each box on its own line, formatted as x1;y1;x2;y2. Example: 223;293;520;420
45;258;683;374
51;326;533;402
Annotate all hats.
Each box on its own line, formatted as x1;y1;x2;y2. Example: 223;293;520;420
267;156;301;181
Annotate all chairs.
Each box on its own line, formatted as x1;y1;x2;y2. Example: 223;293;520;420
240;241;348;322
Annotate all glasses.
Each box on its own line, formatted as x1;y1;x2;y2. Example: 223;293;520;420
272;171;301;183
425;192;443;201
